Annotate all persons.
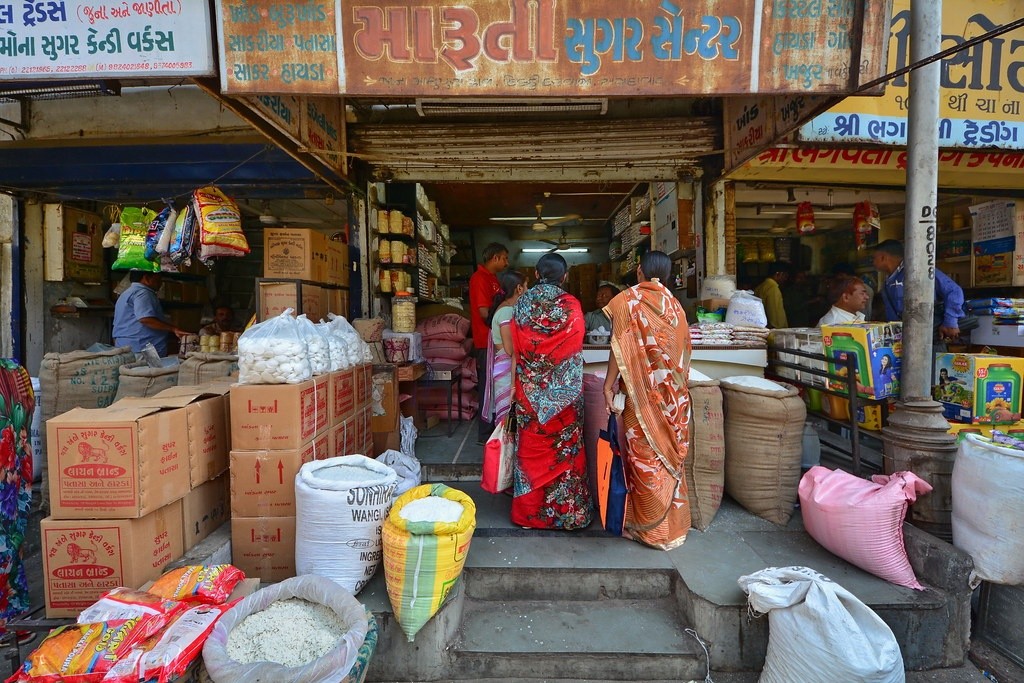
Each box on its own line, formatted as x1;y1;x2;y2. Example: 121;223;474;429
877;354;898;393
601;251;693;553
815;273;869;326
469;241;510;447
884;327;894;347
863;238;966;389
484;269;528;429
753;259;789;328
198;303;236;336
113;270;195;356
510;253;594;526
894;326;901;342
583;283;620;331
938;367;967;397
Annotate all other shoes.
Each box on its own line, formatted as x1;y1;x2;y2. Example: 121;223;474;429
477;439;486;446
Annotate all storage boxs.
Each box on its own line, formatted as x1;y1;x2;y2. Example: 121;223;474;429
39;224;422;619
769;321;1024;446
520;261;611;313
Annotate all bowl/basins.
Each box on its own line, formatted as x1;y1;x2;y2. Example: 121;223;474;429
587;335;610;344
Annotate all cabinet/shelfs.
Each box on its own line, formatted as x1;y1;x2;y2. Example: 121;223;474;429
602;181;656;286
897;197;976;287
377;180;451;303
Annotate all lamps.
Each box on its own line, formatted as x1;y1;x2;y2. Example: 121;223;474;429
786;188;795;203
416;95;608;118
0;79;122;103
756;205;854;219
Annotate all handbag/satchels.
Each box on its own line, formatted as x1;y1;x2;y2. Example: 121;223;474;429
480;421;515;494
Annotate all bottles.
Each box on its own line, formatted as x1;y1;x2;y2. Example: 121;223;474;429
1006;429;1024;441
956;429;987;445
976;362;1021;425
825;332;870;398
391;292;416;333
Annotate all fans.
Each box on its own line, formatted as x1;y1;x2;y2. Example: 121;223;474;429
239;198;324;224
490;203;581;232
538;232;592;252
